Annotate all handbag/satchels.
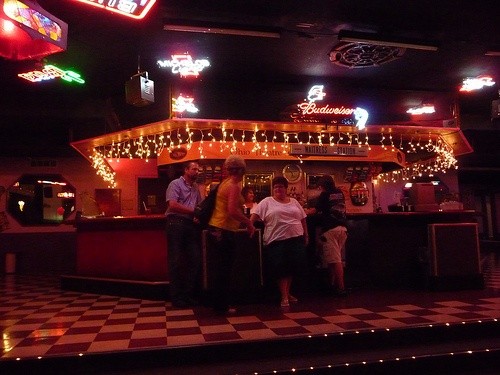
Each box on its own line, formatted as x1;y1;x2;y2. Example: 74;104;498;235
195;183;219;226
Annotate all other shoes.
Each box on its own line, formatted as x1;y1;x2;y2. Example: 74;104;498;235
280;299;290;306
288;296;297;301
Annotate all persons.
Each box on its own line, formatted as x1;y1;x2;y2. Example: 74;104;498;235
162;154;313;317
308;174;350;296
